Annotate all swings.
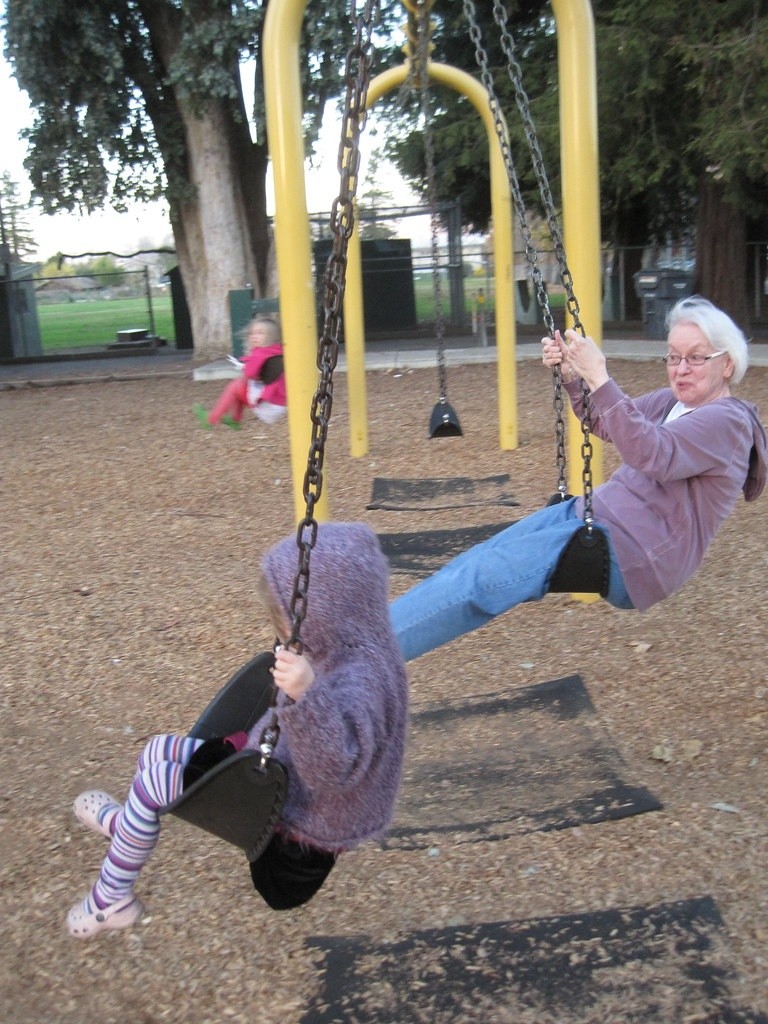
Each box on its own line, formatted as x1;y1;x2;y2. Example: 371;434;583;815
169;0;615;865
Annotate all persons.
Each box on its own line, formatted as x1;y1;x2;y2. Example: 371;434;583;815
368;290;756;665
192;314;287;434
61;512;410;942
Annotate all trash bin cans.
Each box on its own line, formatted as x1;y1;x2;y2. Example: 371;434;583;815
632;268;697;341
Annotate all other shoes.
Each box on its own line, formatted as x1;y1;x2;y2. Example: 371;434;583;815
220;415;241;430
194;402;213;430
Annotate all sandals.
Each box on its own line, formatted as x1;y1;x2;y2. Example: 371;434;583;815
73;790;123;839
66;890;144;939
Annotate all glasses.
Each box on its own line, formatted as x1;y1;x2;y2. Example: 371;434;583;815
663;350;727;366
